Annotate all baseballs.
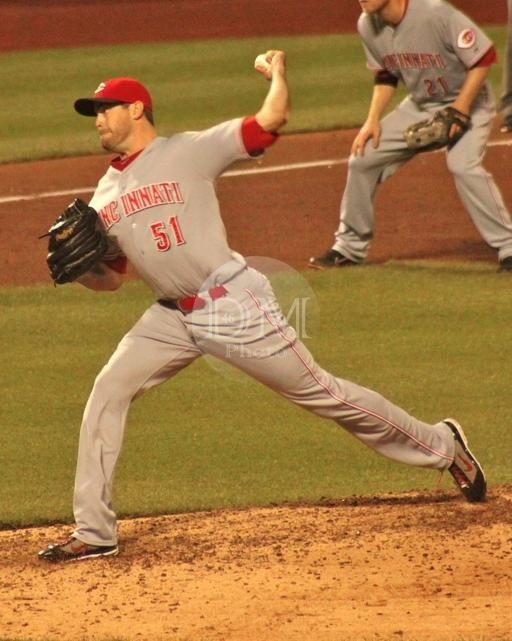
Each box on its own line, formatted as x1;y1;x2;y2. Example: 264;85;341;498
255;53;271;70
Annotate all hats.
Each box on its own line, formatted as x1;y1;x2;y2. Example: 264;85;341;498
74;77;152;117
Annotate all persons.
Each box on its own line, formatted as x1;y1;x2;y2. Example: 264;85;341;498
37;50;485;561
305;1;512;271
500;0;512;133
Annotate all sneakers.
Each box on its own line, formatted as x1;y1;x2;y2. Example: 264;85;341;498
442;418;487;503
500;255;512;273
38;536;119;564
308;249;354;270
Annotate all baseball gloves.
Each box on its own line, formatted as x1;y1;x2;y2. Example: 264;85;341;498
38;199;106;287
407;104;469;156
